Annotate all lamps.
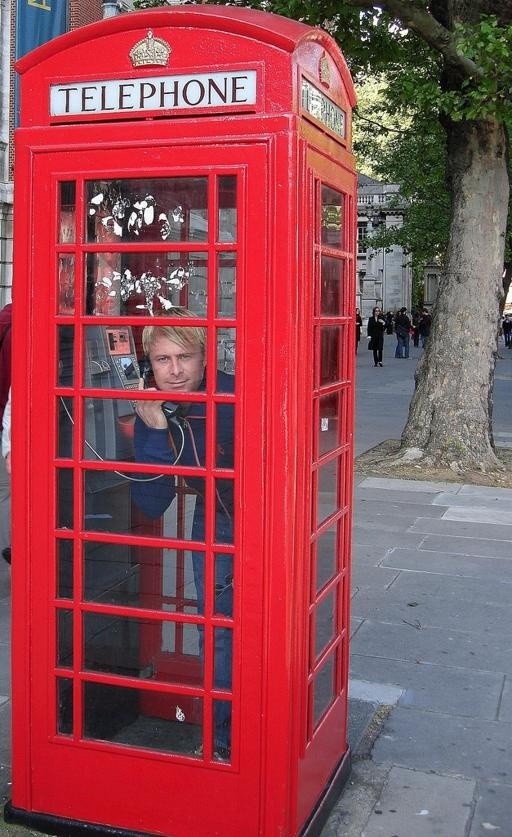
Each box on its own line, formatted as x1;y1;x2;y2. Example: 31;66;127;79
366;204;377;222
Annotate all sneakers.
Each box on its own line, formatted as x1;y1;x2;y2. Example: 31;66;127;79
374;362;384;367
395;356;409;359
505;344;512;349
414;344;424;348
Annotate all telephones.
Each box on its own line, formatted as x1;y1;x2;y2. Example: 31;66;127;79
138;356;181;420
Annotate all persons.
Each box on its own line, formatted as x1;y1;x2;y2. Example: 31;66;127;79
355;305;432;368
128;304;234;767
2;385;13;474
498;312;511;349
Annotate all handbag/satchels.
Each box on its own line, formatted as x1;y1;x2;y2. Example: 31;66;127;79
368;337;374;350
